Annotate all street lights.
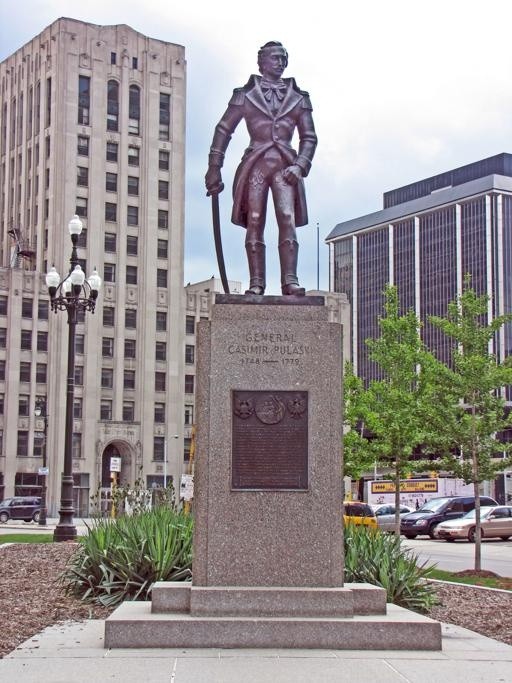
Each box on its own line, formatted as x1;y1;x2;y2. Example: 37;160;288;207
46;210;102;543
34;392;47;527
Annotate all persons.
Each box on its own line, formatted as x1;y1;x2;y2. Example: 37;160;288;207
205;41;318;298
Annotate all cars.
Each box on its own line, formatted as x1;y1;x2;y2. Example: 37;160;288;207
432;505;512;543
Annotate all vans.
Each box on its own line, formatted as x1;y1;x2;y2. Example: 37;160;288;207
369;503;417;535
343;501;377;541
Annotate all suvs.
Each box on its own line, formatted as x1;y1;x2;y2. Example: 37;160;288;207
400;495;499;540
0;496;42;523
